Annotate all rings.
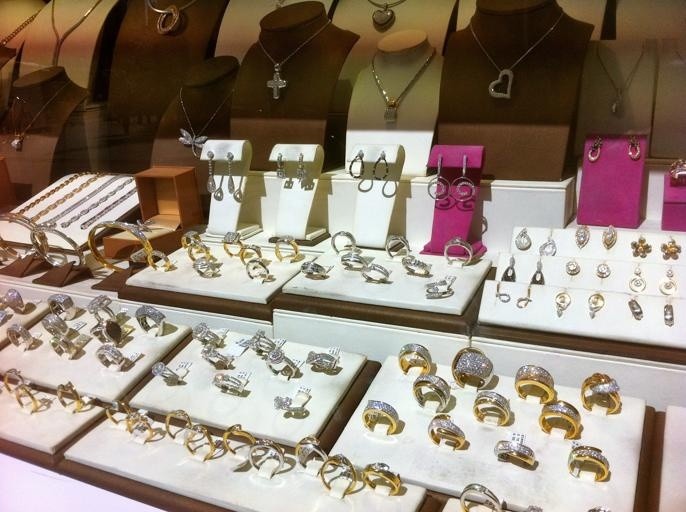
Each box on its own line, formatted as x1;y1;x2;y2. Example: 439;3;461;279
148;231;220;278
411;373;452;413
386;235;410;257
567;443;612;482
222;232;327;282
107;400;400;500
427;414;466;451
331;230;389;282
520;501;545;512
401;255;431;275
579;371;622;416
398;342;435;379
493;438;536;467
474;392;512;429
590;504;613;512
444;236;473;266
1;288;338;415
513;363;557;407
451;344;494;390
424;280;454;298
539;399;583;441
459;481;501;511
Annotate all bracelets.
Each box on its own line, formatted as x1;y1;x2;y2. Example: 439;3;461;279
29;224;85;274
87;221;153;274
0;212;48;266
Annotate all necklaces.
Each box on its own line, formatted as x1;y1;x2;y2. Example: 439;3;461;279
12;171;141;230
10;76;77;154
0;9;39;51
177;83;238;159
365;42;438;130
146;0;196;37
48;0;99;75
465;19;568;102
366;1;405;29
253;20;333;110
590;37;650;121
